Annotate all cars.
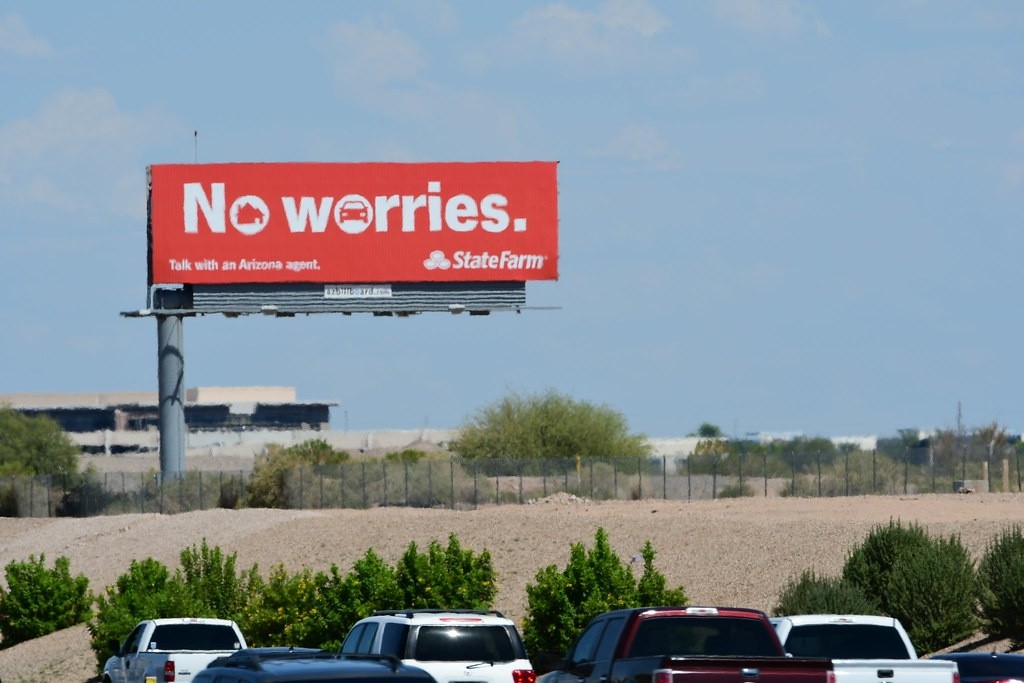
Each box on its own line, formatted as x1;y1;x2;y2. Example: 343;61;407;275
926;652;1024;683
188;646;437;683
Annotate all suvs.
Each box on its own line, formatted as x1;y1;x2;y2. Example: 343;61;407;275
331;606;536;683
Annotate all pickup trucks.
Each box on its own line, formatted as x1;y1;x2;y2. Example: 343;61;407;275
534;605;837;683
99;617;249;683
769;614;963;683
